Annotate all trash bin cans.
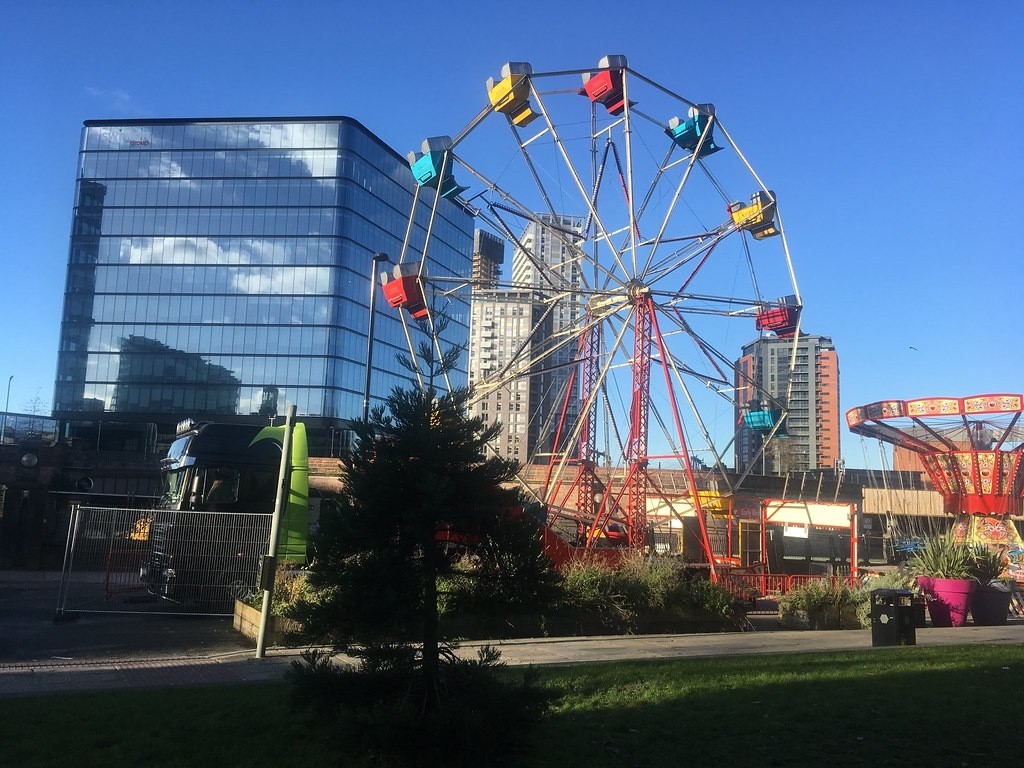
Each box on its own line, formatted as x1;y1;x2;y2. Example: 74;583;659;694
868;587;918;647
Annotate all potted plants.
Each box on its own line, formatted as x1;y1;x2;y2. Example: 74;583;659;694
840;571;913;630
774;570;850;629
968;545;1016;625
909;529;987;627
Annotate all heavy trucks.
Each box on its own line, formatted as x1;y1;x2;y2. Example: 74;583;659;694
138;417;314;608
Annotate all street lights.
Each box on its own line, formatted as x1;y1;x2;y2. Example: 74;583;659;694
1;376;14;443
363;253;388;422
